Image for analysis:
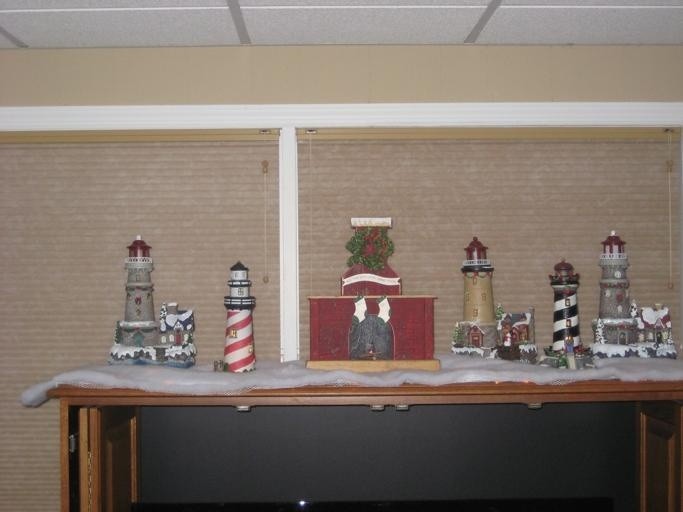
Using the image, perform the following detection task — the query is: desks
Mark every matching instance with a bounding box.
[45,379,683,512]
